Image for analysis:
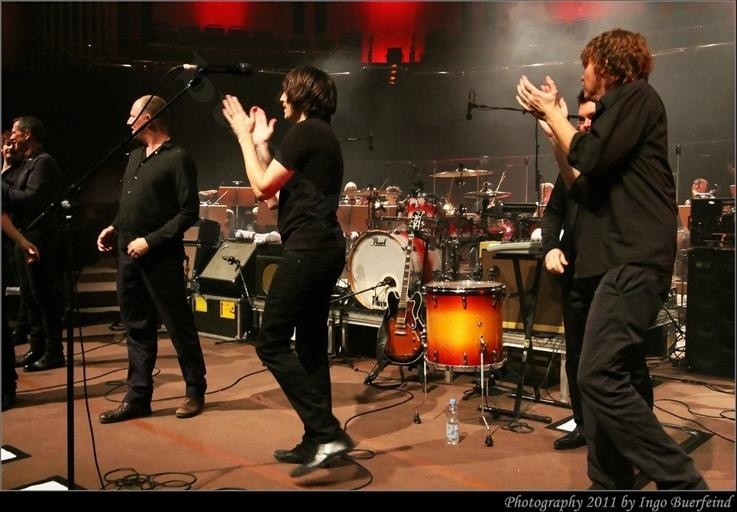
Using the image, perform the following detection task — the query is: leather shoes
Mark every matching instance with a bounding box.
[274,443,329,468]
[99,401,151,423]
[553,431,587,450]
[12,331,27,346]
[15,349,37,367]
[23,353,65,372]
[176,397,204,417]
[289,434,355,477]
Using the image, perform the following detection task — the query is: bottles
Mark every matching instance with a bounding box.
[447,399,459,445]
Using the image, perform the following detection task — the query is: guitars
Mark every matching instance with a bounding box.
[377,210,425,365]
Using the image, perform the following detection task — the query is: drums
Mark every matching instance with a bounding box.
[477,212,543,242]
[441,216,479,241]
[422,281,508,372]
[400,195,446,229]
[348,229,443,310]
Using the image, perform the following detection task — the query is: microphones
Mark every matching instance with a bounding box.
[183,62,253,75]
[368,130,374,151]
[466,93,472,120]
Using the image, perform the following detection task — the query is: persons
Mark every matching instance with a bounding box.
[1,116,66,412]
[675,162,735,313]
[340,181,557,246]
[96,95,207,424]
[542,89,597,450]
[514,28,709,492]
[197,186,282,245]
[220,65,354,478]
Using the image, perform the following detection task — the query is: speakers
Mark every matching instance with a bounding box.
[184,217,349,358]
[476,241,564,335]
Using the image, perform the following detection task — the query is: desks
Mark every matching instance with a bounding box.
[493,250,573,424]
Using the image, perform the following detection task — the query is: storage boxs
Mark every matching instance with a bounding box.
[191,292,253,342]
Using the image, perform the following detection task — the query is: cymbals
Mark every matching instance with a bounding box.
[464,190,511,200]
[340,190,404,196]
[430,169,495,177]
